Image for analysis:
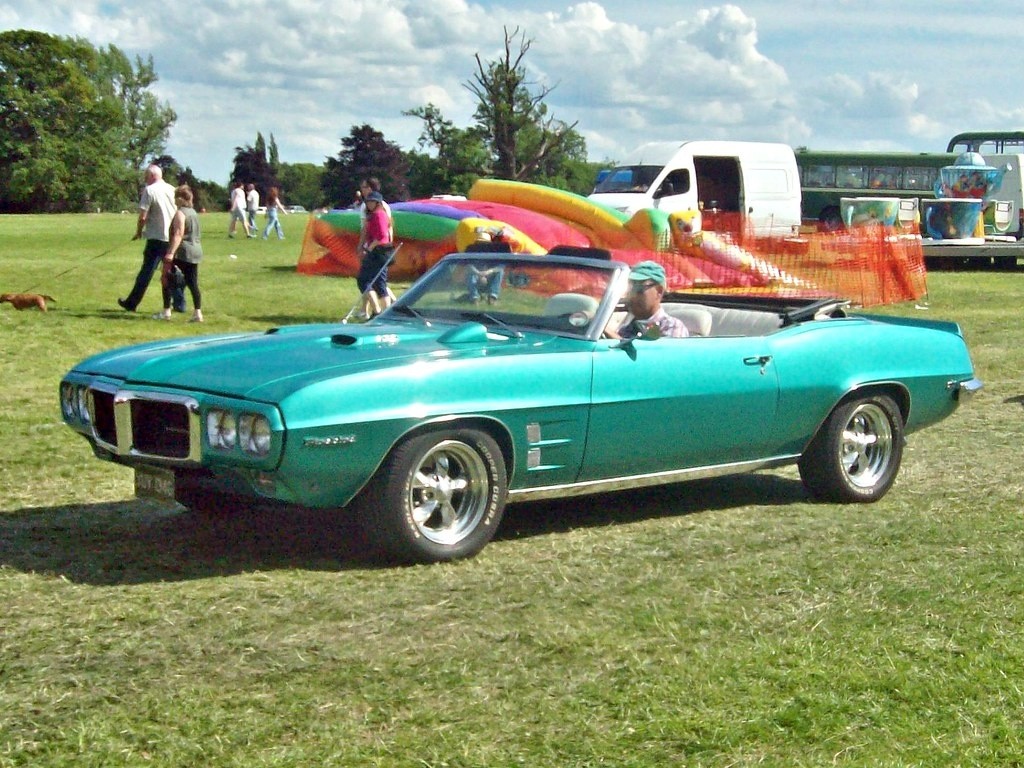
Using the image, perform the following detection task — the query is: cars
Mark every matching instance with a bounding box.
[57,239,985,559]
[256,207,267,215]
[284,205,310,214]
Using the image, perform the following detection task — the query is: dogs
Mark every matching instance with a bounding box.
[0,292,56,314]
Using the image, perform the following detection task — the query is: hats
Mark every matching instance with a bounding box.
[475,232,492,243]
[629,260,666,288]
[364,191,383,202]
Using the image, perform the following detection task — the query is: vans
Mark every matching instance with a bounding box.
[981,153,1024,238]
[430,195,466,202]
[582,139,805,253]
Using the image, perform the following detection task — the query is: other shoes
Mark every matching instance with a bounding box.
[118,297,135,312]
[354,311,369,320]
[228,234,234,238]
[246,235,254,237]
[152,312,170,320]
[186,316,203,322]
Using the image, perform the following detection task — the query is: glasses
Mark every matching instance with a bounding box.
[633,283,657,294]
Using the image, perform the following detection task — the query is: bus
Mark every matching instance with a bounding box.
[792,148,964,233]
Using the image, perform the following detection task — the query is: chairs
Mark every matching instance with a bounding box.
[543,293,599,319]
[667,309,711,337]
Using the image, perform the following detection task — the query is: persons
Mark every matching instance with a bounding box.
[346,177,396,320]
[228,182,258,238]
[464,233,513,307]
[152,185,204,322]
[246,184,259,232]
[356,191,391,326]
[117,164,178,312]
[261,187,287,240]
[582,260,689,338]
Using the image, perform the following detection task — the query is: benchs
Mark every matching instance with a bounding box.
[658,302,785,338]
